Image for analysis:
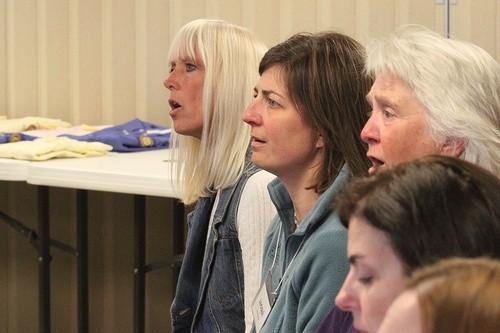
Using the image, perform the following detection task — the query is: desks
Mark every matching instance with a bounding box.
[0,124,186,333]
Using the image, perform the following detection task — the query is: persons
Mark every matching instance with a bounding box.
[333,153,500,333]
[162,18,270,333]
[376,256,500,333]
[242,31,377,333]
[361,24,500,179]
